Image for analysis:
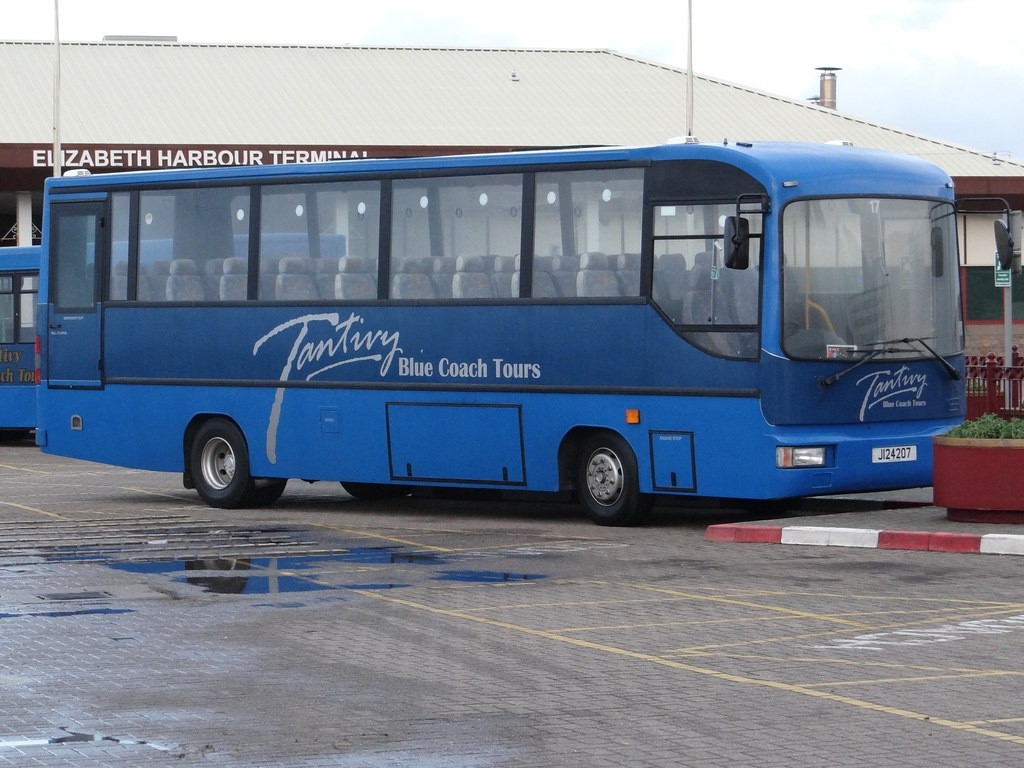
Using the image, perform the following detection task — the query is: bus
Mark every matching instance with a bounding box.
[32,139,1015,528]
[0,234,347,428]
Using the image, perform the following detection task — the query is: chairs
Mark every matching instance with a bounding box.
[114,251,780,368]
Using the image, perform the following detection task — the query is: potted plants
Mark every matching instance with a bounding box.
[932,411,1024,523]
